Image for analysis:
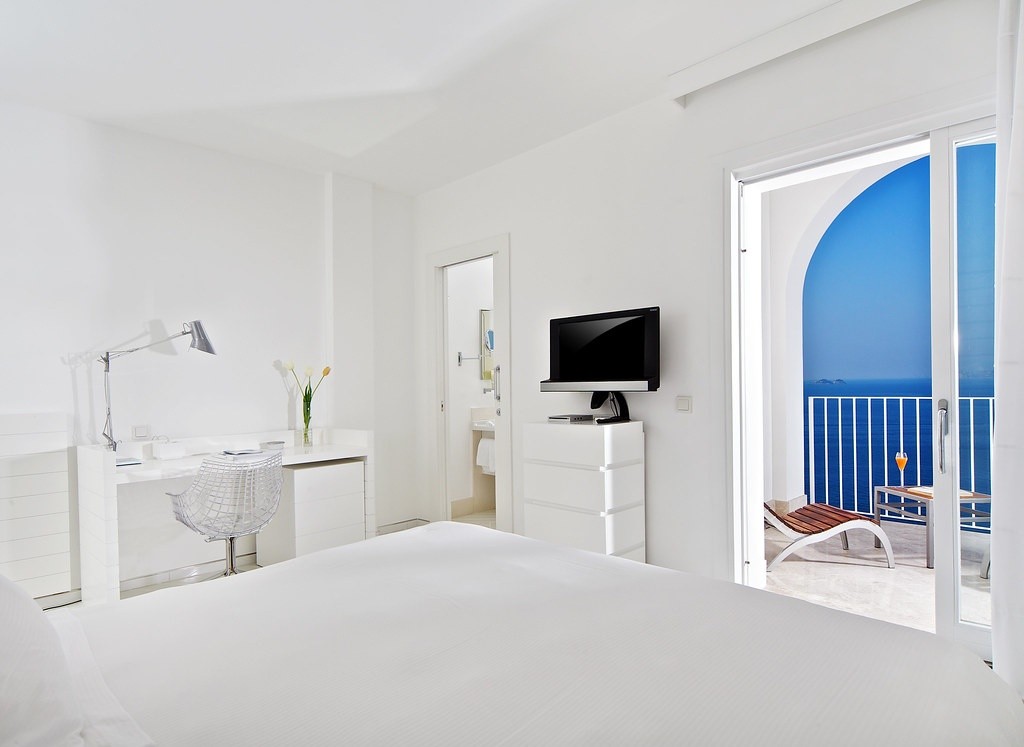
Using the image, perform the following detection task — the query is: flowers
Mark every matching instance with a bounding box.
[283,360,335,442]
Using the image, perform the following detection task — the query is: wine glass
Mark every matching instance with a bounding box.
[895,452,908,488]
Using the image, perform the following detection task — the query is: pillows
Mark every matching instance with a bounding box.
[0,574,156,747]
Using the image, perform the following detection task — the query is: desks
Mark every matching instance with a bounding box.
[77,428,376,606]
[871,485,991,568]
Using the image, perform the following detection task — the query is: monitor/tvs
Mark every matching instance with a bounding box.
[548,306,661,389]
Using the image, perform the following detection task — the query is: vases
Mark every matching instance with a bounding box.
[303,427,313,447]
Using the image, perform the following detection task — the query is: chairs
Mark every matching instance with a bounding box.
[764,501,898,572]
[163,451,286,583]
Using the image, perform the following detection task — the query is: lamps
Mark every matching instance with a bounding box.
[99,316,223,454]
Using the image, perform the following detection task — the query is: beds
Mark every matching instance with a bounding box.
[0,519,1024,747]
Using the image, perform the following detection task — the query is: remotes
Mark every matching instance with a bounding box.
[596,416,632,424]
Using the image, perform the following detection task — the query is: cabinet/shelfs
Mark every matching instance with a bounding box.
[520,417,648,562]
[253,458,364,564]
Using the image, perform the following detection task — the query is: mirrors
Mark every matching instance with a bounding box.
[479,309,494,381]
